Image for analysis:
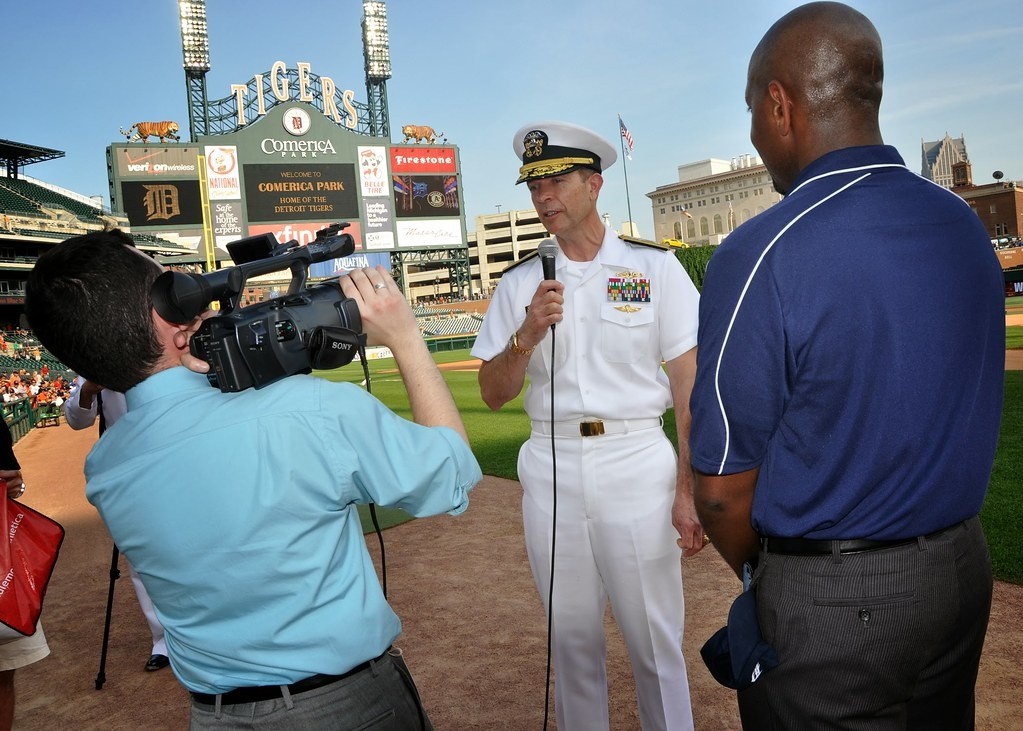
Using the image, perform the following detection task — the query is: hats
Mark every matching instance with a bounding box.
[512,121,618,184]
[10,389,14,393]
[14,381,19,385]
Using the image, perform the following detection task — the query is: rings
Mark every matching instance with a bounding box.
[375,283,385,290]
[703,534,711,546]
[21,484,25,492]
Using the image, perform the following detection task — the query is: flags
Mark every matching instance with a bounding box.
[619,117,634,152]
[683,211,693,218]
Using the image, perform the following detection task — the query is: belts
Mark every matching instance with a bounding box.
[760,533,916,555]
[192,645,396,706]
[531,418,660,437]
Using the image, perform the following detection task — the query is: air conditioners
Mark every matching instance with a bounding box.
[1007,180,1016,189]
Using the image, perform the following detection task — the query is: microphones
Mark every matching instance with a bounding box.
[538,239,559,329]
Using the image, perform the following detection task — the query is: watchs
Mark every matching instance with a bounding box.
[508,333,538,355]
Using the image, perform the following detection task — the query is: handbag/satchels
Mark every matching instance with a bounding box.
[0,478,67,635]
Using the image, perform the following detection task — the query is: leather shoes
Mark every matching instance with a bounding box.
[145,654,170,671]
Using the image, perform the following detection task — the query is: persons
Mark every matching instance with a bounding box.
[0,328,43,361]
[471,121,699,731]
[0,415,24,731]
[477,291,481,299]
[691,0,1006,730]
[4,212,11,229]
[0,365,77,425]
[24,232,486,731]
[418,296,451,307]
